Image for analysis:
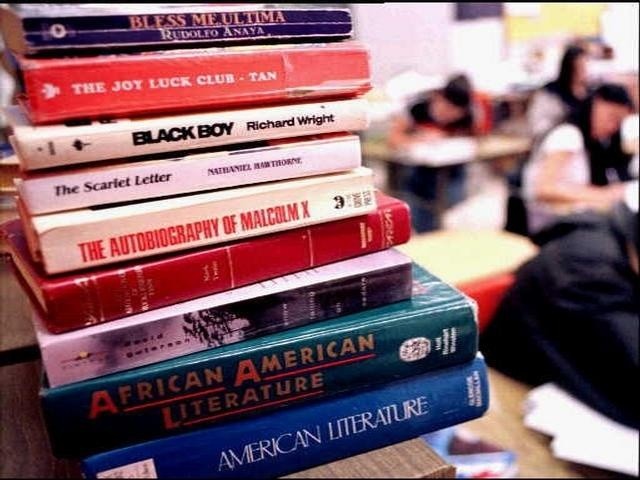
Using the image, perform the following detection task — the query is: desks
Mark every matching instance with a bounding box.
[278,437,456,480]
[364,134,533,226]
[0,260,87,480]
[391,227,540,328]
[427,369,637,480]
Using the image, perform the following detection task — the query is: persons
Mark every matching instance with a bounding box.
[526,47,590,146]
[504,84,639,247]
[478,198,638,429]
